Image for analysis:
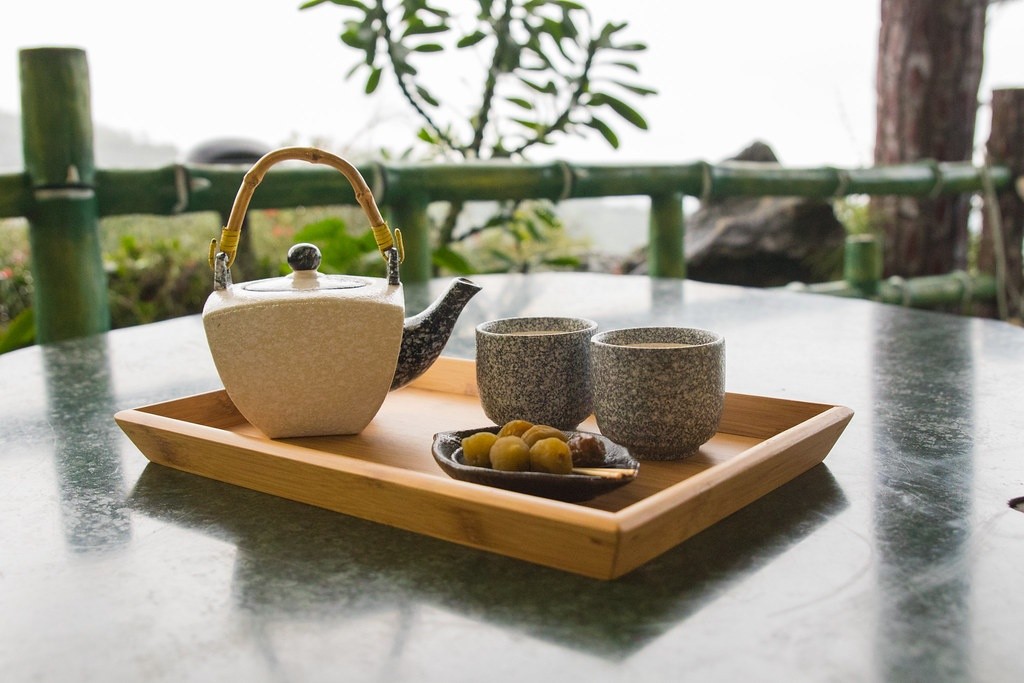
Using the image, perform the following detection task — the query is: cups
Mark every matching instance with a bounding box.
[591,326,726,463]
[475,316,599,431]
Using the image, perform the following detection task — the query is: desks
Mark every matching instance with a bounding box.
[0,269,1024,683]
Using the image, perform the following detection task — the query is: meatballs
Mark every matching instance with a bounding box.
[460,419,607,475]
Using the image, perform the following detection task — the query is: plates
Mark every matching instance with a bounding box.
[431,426,639,504]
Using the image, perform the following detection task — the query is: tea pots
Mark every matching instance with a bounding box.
[201,146,482,440]
